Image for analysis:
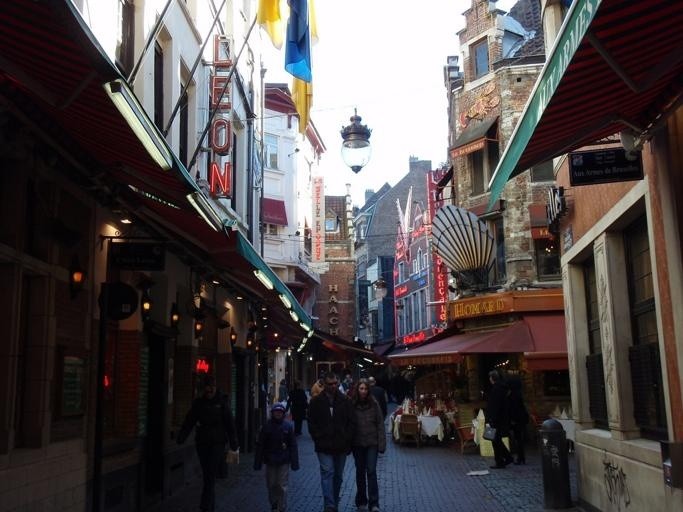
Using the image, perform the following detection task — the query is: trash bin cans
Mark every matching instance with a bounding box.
[538,419,572,510]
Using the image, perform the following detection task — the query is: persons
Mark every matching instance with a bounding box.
[277,374,388,435]
[254,402,299,511]
[350,377,386,511]
[177,380,240,511]
[480,369,514,470]
[507,377,530,464]
[305,372,358,512]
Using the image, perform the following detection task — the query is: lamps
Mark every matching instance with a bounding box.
[171,301,180,327]
[141,290,152,322]
[230,107,373,173]
[230,326,237,344]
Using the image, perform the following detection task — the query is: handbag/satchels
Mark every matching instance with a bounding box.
[226,449,240,465]
[482,421,497,441]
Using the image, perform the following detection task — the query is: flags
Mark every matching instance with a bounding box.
[255,0,322,138]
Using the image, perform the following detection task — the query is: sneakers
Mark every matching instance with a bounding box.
[270,501,380,512]
[489,452,527,469]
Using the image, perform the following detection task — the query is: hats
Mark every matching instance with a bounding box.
[269,403,286,413]
